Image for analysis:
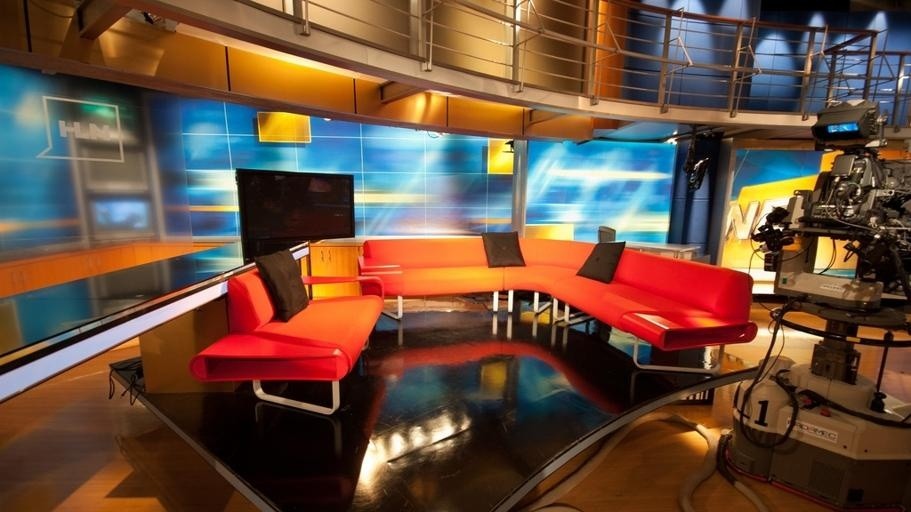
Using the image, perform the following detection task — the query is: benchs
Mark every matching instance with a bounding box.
[188,268,385,416]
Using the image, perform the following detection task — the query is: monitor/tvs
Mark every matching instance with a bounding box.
[237,169,355,265]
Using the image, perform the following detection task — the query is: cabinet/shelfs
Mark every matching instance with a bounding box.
[310,241,364,301]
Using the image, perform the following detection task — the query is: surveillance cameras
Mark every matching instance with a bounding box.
[682,135,710,193]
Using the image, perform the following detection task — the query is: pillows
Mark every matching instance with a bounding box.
[481,232,526,267]
[254,249,309,322]
